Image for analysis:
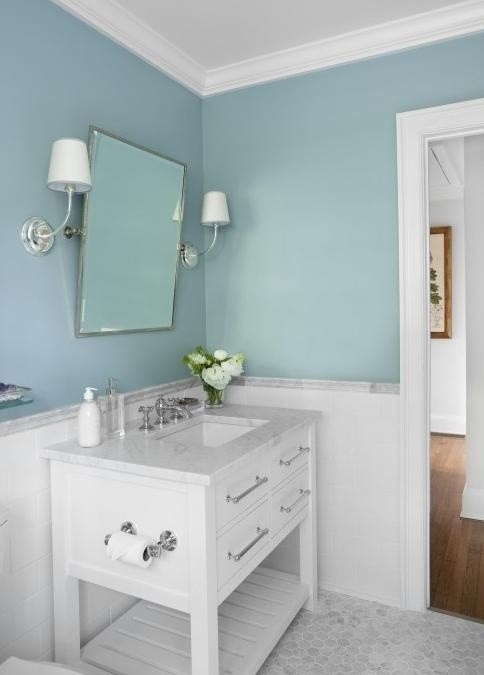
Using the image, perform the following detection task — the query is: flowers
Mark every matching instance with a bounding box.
[181,345,247,403]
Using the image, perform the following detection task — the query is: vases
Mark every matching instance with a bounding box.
[201,380,224,410]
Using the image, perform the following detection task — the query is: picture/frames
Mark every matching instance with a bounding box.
[429,228,454,342]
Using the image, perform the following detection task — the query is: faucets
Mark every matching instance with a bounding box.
[154,398,192,425]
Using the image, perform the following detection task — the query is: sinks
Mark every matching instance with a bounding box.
[141,413,272,449]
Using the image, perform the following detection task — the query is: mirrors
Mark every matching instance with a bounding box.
[78,123,187,338]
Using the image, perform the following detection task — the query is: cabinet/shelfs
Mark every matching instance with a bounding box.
[38,403,323,675]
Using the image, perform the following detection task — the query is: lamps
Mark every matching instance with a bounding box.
[180,190,230,271]
[20,137,92,255]
